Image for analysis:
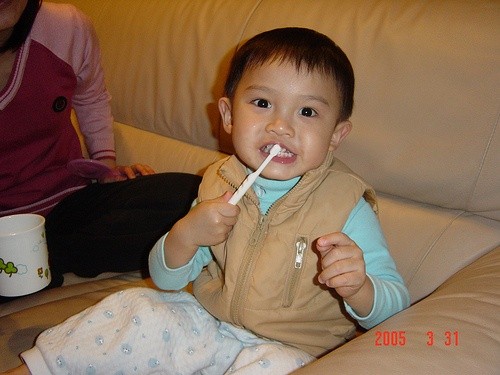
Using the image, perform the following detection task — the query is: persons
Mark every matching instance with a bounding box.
[0,0,202,302]
[0,27,411,375]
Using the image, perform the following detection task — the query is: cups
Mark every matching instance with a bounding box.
[0,214,53,298]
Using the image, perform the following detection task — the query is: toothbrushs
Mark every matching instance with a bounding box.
[227,144,282,207]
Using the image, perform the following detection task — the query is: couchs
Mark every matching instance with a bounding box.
[0,0,499,373]
